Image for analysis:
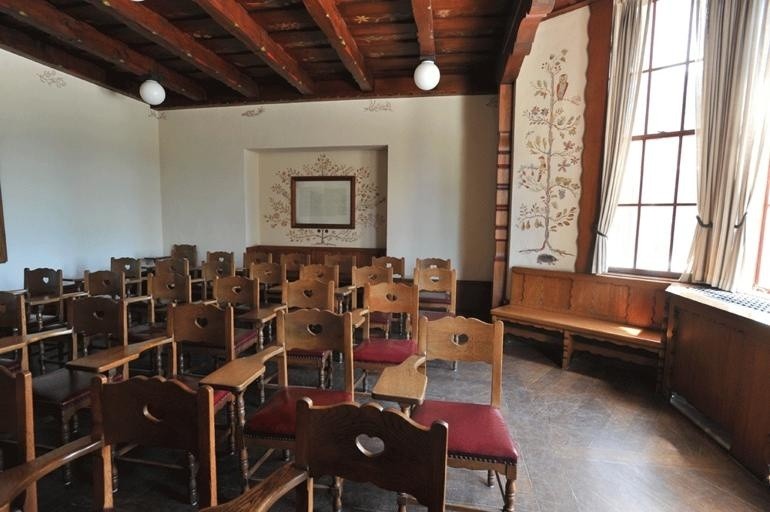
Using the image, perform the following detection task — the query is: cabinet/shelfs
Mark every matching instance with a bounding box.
[664,284,770,493]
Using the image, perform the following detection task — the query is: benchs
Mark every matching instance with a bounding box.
[487,264,674,373]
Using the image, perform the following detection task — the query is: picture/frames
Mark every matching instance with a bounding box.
[290,175,356,230]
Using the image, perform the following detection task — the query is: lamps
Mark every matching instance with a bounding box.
[140,69,168,106]
[413,53,441,90]
